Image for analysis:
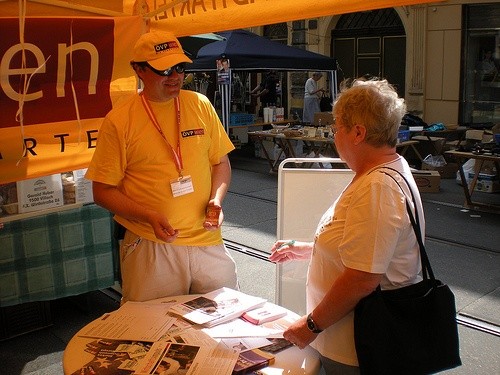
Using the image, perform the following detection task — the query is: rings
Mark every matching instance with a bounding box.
[294,344,296,346]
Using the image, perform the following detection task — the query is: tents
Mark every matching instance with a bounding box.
[184,29,337,134]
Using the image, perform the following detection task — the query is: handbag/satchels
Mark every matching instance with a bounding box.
[321,92,332,111]
[354,279,461,375]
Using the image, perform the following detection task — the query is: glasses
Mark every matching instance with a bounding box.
[143,62,186,77]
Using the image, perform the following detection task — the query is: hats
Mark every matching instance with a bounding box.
[129,32,193,70]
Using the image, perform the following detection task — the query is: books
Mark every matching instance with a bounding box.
[233,349,275,375]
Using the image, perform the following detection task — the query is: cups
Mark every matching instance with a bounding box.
[324,132,329,137]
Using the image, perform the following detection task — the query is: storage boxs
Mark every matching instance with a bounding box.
[230,107,333,162]
[398,124,500,194]
[0,300,57,343]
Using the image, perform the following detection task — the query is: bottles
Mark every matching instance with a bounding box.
[264,102,284,123]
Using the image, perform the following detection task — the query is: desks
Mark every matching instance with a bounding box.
[230,120,291,128]
[250,130,424,174]
[63,293,322,375]
[425,125,468,156]
[443,149,500,210]
[0,203,121,309]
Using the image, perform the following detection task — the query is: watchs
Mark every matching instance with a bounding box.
[306,313,323,333]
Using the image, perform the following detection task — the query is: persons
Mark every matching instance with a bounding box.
[303,72,324,123]
[269,77,426,375]
[84,31,237,305]
[220,60,229,73]
[155,357,192,375]
[249,72,277,107]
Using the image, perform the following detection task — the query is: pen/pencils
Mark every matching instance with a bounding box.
[278,240,295,249]
